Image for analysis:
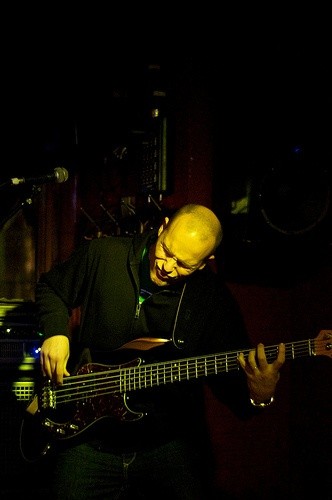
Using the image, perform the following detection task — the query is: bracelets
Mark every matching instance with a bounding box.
[248,394,274,409]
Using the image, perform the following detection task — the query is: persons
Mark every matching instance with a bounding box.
[34,203,287,500]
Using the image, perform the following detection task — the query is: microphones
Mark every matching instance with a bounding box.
[7,167,68,187]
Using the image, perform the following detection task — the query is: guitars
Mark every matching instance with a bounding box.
[20,329,332,464]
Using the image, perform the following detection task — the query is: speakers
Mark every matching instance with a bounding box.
[203,0,330,257]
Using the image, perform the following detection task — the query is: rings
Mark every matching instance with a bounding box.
[251,366,258,371]
